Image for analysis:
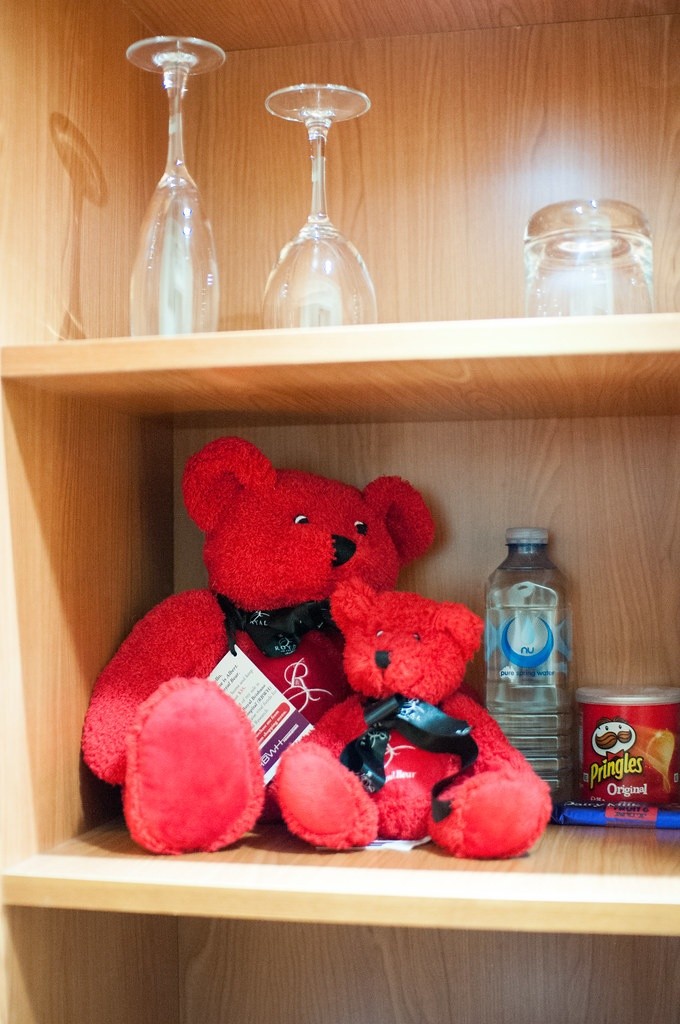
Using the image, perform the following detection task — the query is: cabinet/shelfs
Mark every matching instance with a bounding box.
[0,0,680,1024]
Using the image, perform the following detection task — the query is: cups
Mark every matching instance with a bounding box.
[526,200,654,313]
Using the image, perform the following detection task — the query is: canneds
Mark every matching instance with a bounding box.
[573,683,680,809]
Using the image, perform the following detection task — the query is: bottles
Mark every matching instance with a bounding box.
[484,528,575,816]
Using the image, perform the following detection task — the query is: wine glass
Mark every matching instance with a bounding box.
[126,37,226,335]
[264,84,377,328]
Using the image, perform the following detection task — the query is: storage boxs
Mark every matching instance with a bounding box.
[576,686,680,808]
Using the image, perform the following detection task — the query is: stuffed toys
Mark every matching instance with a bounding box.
[81,434,437,845]
[270,577,554,859]
